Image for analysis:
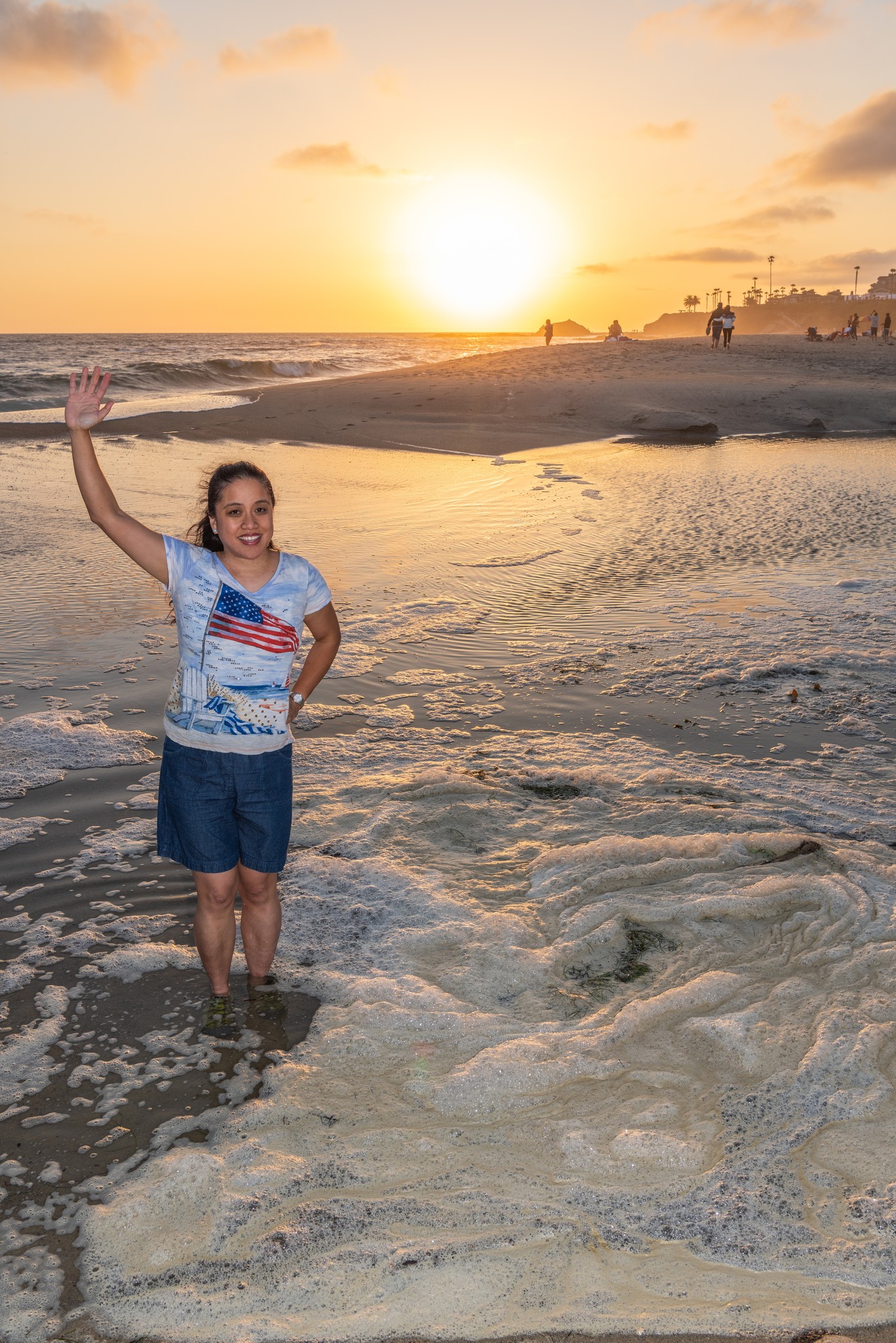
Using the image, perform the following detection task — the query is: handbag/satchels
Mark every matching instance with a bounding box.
[732,325,735,330]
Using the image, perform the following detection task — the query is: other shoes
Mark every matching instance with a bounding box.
[706,327,711,335]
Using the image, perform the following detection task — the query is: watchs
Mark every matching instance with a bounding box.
[288,691,304,709]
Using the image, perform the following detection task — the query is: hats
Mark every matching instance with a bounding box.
[717,302,723,307]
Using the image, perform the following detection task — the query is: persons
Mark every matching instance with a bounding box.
[881,313,891,342]
[544,319,553,346]
[63,365,342,994]
[707,302,735,349]
[847,313,860,341]
[870,309,879,341]
[713,305,736,349]
[605,320,624,341]
